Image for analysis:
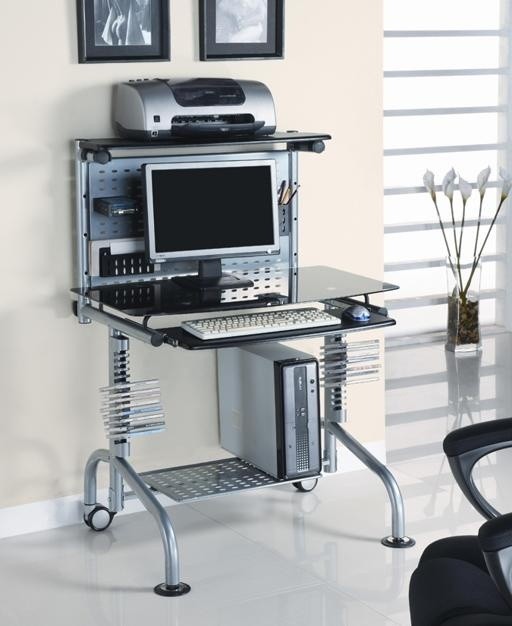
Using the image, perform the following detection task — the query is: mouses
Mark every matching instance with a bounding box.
[345,302,371,323]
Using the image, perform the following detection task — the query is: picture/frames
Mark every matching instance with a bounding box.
[76,0,285,64]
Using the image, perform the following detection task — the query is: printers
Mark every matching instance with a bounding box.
[109,76,277,143]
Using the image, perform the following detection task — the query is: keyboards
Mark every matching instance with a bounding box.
[182,307,342,341]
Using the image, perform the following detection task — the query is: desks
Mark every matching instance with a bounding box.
[72,263,415,596]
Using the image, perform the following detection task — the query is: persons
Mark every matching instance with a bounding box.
[104,0,141,45]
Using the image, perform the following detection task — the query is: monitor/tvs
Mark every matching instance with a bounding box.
[142,159,282,287]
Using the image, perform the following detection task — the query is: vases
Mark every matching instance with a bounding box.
[446,289,483,354]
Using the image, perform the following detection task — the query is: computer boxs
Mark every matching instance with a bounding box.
[212,343,322,481]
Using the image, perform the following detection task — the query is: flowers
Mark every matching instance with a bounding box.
[423,166,512,301]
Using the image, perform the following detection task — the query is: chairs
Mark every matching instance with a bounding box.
[409,417,512,626]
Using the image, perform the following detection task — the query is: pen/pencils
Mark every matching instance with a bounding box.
[278,180,300,206]
